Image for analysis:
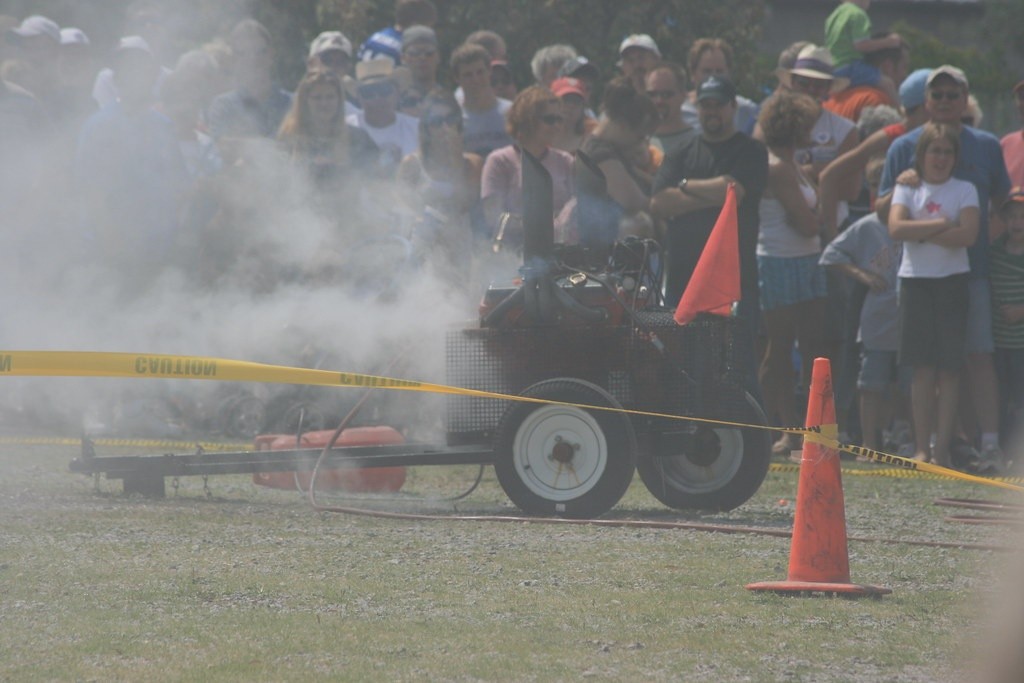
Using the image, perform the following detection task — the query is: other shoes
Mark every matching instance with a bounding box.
[977,444,1004,476]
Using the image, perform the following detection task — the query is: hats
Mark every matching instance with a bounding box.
[619,33,662,59]
[310,31,352,58]
[998,185,1024,214]
[9,16,61,43]
[61,28,90,46]
[899,68,933,110]
[695,75,735,104]
[571,56,598,79]
[776,43,850,96]
[121,36,150,54]
[341,60,414,100]
[925,64,969,88]
[552,78,587,100]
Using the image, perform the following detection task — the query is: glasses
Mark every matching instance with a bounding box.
[406,47,437,58]
[428,115,460,128]
[930,89,963,103]
[356,84,390,100]
[539,114,565,126]
[646,90,675,99]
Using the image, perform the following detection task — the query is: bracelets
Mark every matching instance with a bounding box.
[680,177,687,190]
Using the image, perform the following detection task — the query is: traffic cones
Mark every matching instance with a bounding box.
[745,354,899,596]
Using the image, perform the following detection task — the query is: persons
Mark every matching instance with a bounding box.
[0,0,1023,472]
[650,73,768,385]
[888,120,980,471]
